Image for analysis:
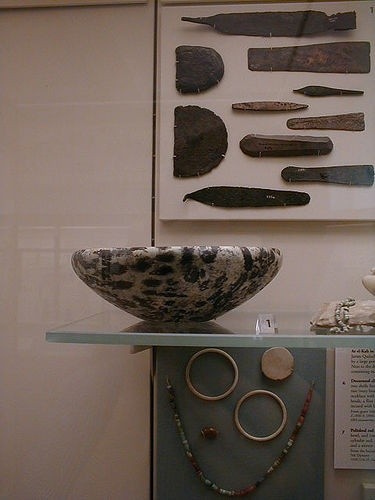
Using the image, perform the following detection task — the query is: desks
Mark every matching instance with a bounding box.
[45,309,375,349]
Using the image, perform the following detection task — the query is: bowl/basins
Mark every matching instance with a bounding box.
[71,246,283,323]
